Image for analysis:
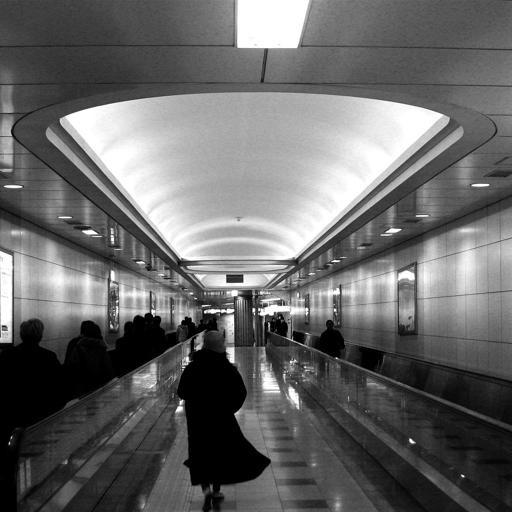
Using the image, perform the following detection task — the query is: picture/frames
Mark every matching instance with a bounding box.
[398,262,418,336]
[0,247,15,347]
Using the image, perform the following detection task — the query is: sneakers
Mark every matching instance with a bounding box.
[202,492,224,512]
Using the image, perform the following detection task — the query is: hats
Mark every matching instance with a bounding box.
[203,331,226,354]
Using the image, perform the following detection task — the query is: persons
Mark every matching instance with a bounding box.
[319,320,345,358]
[0,319,63,512]
[63,313,218,398]
[264,314,288,338]
[177,330,271,512]
